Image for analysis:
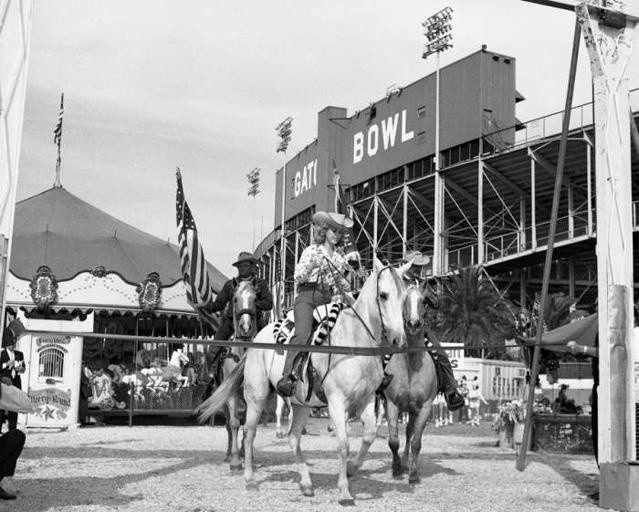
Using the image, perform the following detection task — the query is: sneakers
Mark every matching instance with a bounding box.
[1,487,17,501]
[277,376,294,397]
[444,390,465,411]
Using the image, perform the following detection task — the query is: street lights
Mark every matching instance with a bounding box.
[275,116,292,320]
[246,165,259,256]
[422,6,454,280]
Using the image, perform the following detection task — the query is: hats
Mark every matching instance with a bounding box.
[232,252,258,268]
[312,211,354,231]
[404,250,430,266]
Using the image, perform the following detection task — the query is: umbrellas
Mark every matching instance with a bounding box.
[53,108,65,145]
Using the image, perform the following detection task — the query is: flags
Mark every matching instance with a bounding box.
[174,179,221,332]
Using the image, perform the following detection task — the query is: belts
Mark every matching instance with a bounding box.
[301,282,333,291]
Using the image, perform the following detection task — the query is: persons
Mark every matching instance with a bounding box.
[276,211,355,395]
[1,337,26,435]
[203,250,274,371]
[457,374,490,427]
[0,317,30,498]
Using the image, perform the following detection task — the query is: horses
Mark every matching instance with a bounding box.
[193,254,416,507]
[382,273,439,486]
